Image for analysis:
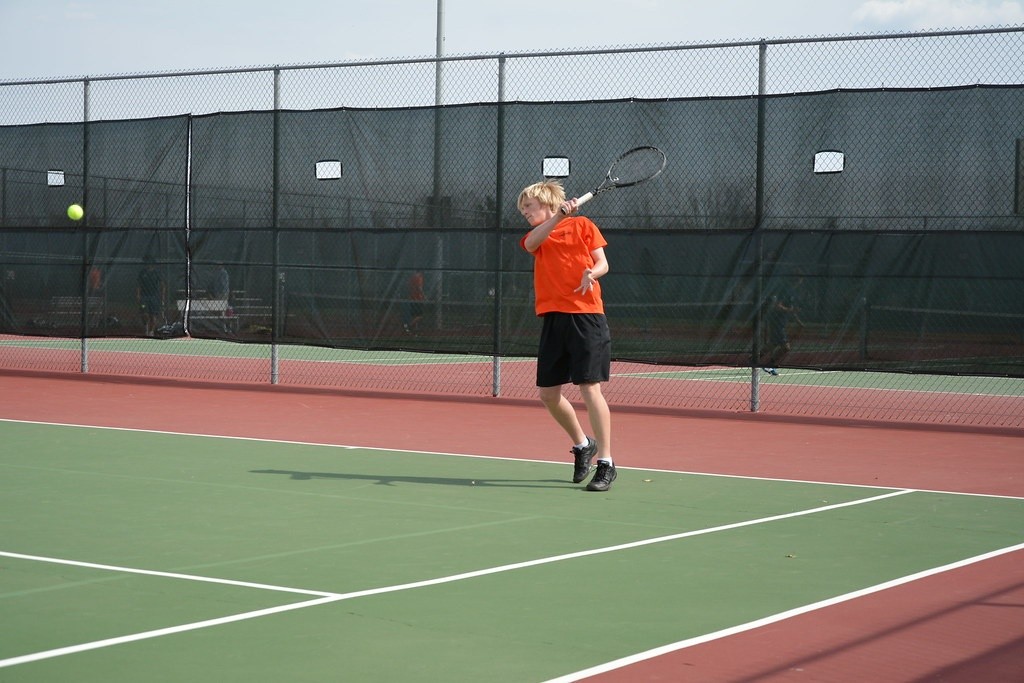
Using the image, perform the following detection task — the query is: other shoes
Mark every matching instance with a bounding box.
[403,322,411,336]
[415,333,422,338]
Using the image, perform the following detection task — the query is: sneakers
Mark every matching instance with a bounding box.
[569,435,598,483]
[763,367,778,376]
[586,460,617,491]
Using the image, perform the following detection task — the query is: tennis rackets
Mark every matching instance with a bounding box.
[561,145,666,216]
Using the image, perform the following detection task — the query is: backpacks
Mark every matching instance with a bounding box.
[153,319,186,341]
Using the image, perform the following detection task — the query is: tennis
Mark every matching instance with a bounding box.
[67,205,84,220]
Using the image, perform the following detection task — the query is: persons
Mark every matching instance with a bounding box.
[91,265,107,295]
[517,180,617,490]
[137,256,166,337]
[214,264,230,303]
[403,267,426,338]
[756,247,804,367]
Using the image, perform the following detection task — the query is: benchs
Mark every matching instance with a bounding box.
[35,295,103,334]
[174,290,239,336]
